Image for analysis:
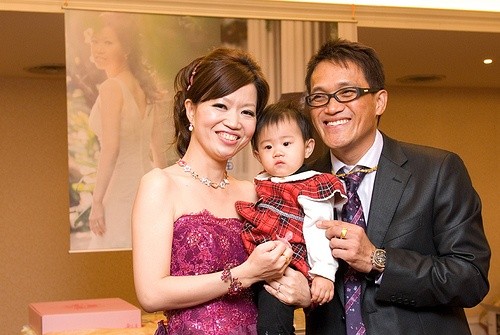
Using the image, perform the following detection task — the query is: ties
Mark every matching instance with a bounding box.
[337,166,370,334]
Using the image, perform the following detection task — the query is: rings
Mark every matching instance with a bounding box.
[282,252,290,264]
[276,283,283,292]
[340,227,348,239]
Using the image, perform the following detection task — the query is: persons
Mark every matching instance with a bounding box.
[88,13,165,251]
[297,39,492,335]
[132,50,312,334]
[236,101,346,334]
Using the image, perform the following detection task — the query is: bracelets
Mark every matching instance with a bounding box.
[220,259,250,296]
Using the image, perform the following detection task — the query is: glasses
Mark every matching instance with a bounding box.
[305,86,383,107]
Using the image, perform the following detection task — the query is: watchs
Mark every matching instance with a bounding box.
[364,247,387,287]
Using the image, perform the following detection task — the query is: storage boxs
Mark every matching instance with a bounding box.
[28,297,142,335]
